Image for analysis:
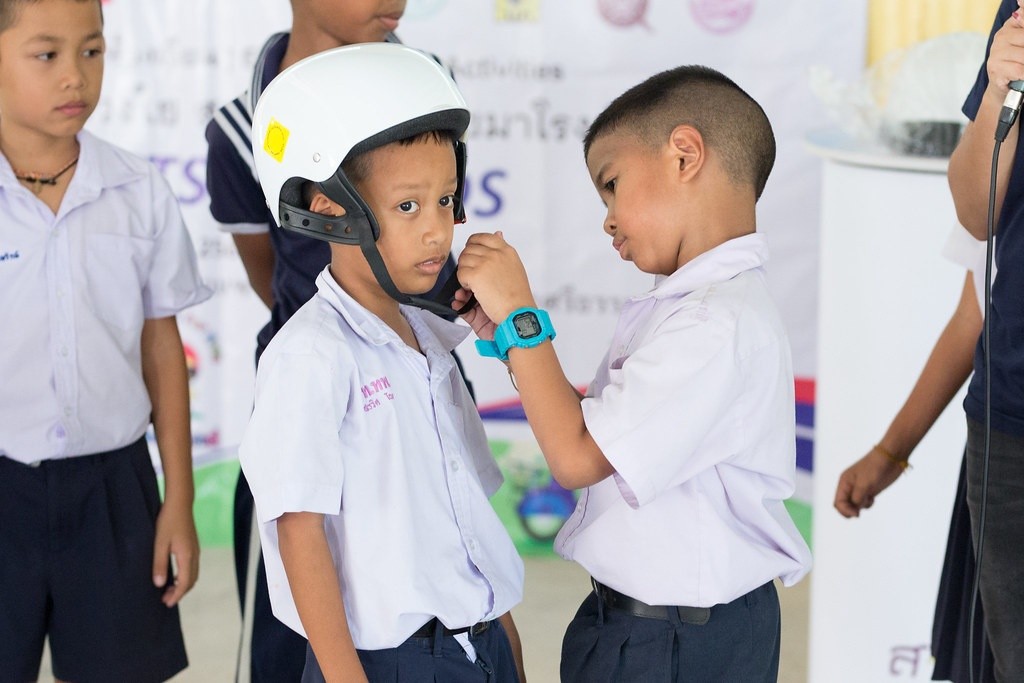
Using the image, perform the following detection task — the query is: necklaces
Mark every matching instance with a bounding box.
[17,153,78,193]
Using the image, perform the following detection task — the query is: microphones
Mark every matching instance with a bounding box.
[995,80,1024,142]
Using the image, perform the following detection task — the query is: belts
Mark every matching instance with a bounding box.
[590,575,710,626]
[409,617,490,639]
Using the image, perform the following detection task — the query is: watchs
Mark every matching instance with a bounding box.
[476,307,554,360]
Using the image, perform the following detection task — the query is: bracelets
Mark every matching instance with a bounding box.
[875,444,914,469]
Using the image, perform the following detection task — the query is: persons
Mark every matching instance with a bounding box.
[0,1,217,683]
[203,0,477,683]
[830,0,1024,683]
[450,62,814,683]
[238,41,530,683]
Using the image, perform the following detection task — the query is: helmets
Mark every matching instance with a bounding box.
[251,41,471,228]
